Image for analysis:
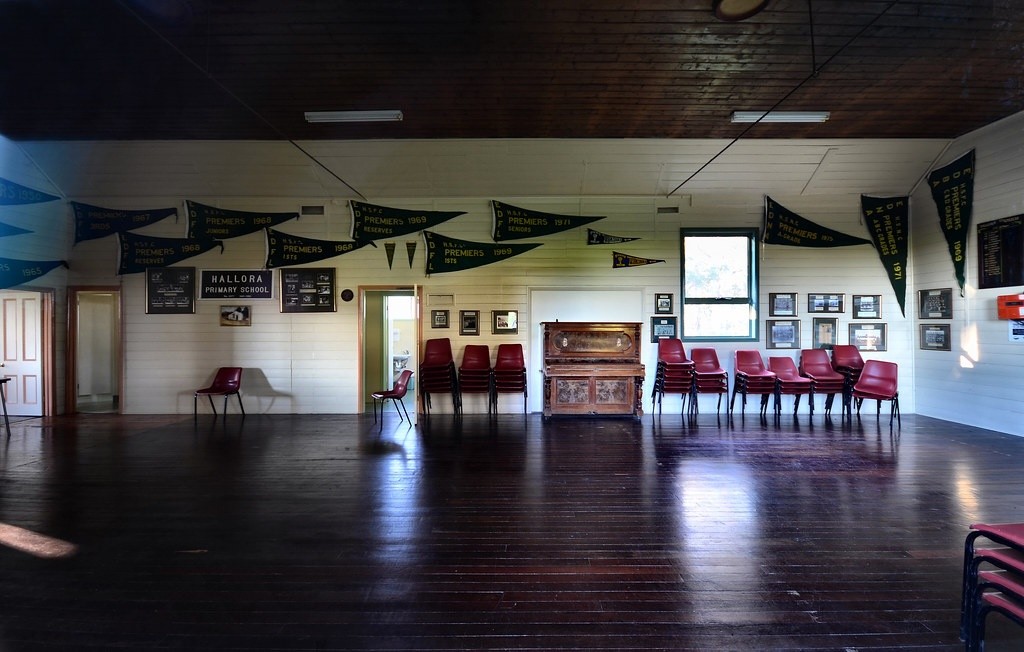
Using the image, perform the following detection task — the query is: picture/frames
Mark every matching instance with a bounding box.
[919,323,952,352]
[917,288,953,318]
[491,309,519,335]
[808,292,846,314]
[852,294,882,319]
[655,292,675,314]
[650,315,679,343]
[849,323,888,351]
[220,305,252,327]
[431,309,451,328]
[145,266,196,314]
[458,309,481,335]
[279,267,338,314]
[767,320,801,349]
[769,293,798,317]
[813,317,838,350]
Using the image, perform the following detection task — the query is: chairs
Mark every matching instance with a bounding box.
[372,370,414,427]
[955,522,1023,652]
[195,367,244,421]
[653,338,902,428]
[420,338,528,425]
